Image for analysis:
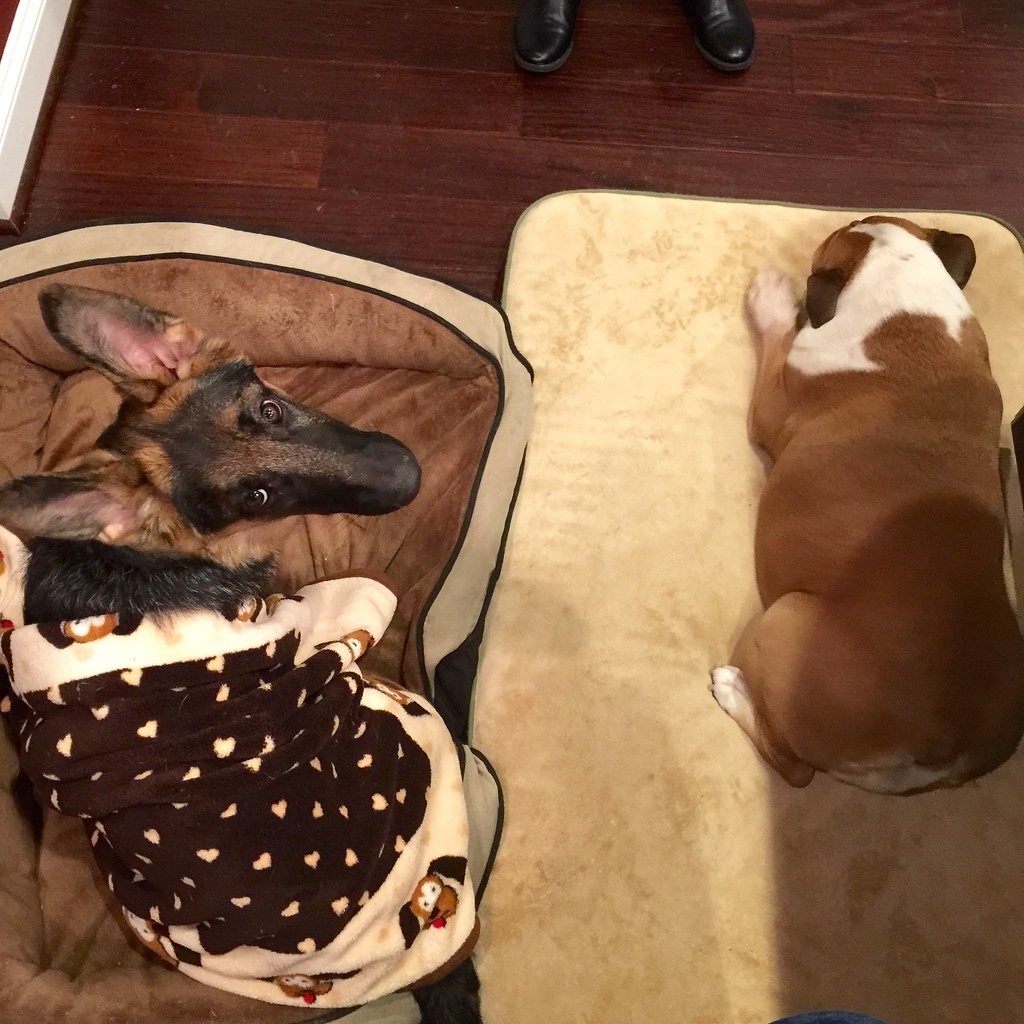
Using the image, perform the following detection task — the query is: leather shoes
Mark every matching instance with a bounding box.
[511,0,580,73]
[681,0,756,71]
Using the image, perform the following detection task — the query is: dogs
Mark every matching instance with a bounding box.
[0,285,423,626]
[703,212,1024,820]
